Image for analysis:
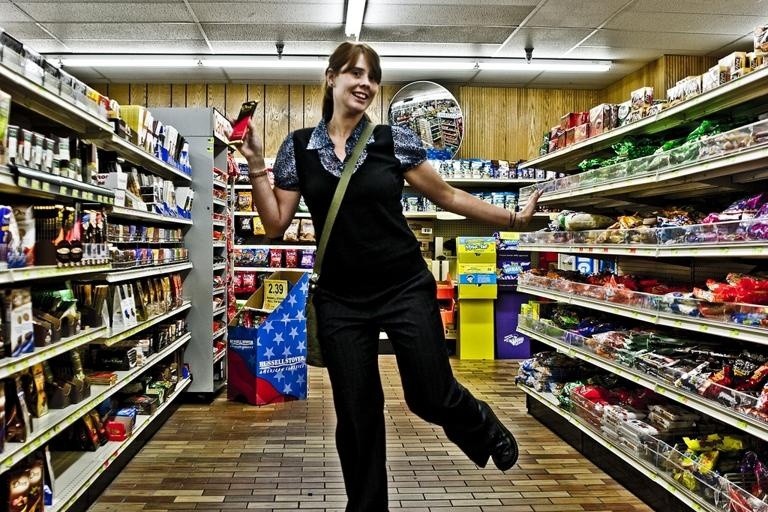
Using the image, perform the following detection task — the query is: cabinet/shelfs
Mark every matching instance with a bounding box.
[517,61,768,511]
[233,155,552,347]
[391,99,460,149]
[0,28,229,511]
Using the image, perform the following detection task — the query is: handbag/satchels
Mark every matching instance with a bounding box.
[306,283,326,368]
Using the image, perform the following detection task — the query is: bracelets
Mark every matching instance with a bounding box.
[250,167,269,179]
[509,210,517,229]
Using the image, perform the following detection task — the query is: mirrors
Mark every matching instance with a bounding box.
[387,80,466,160]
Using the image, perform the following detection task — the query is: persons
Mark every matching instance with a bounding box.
[222,41,549,511]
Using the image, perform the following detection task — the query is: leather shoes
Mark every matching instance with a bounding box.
[480,400,518,470]
